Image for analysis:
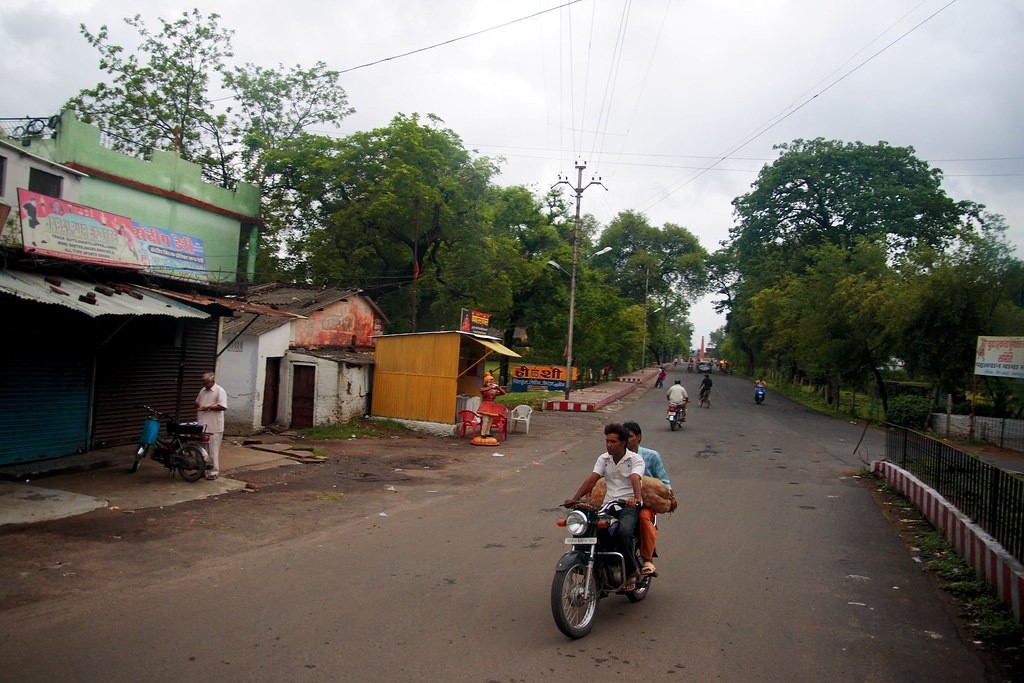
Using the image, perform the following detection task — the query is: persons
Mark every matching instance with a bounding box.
[623,420,673,575]
[689,358,694,363]
[655,369,666,387]
[666,379,689,422]
[699,374,713,398]
[564,423,646,592]
[674,356,678,366]
[192,371,228,480]
[461,312,472,332]
[755,376,766,387]
[600,365,610,383]
[476,371,505,439]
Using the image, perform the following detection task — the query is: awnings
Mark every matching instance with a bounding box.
[0,262,214,321]
[456,334,523,379]
[131,278,311,358]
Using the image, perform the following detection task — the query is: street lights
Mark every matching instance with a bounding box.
[662,318,675,363]
[641,307,662,374]
[670,333,682,362]
[548,247,614,400]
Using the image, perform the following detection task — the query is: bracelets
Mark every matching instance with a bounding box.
[208,408,210,411]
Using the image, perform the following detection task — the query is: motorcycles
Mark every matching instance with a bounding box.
[665,398,691,432]
[549,496,660,640]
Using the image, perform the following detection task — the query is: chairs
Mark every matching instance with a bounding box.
[459,401,533,444]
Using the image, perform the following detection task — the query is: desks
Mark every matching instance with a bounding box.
[456,396,481,423]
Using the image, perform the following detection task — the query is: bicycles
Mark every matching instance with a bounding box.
[131,404,214,482]
[697,385,712,409]
[657,373,664,389]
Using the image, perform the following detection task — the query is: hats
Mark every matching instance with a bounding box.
[703,374,709,377]
[675,379,681,384]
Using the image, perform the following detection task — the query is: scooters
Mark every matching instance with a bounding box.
[687,362,695,373]
[752,384,766,405]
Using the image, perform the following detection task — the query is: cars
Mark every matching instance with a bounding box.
[697,361,713,374]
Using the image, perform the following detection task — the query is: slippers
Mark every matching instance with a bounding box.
[641,561,656,574]
[621,578,637,592]
